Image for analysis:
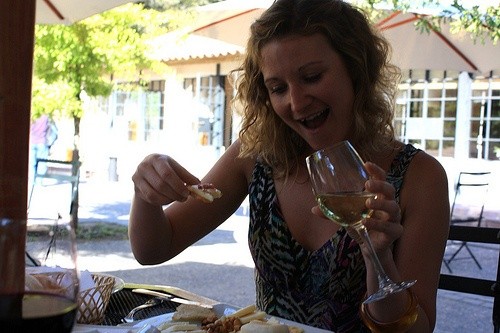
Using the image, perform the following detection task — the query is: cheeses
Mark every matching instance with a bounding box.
[229,303,266,323]
[161,321,208,333]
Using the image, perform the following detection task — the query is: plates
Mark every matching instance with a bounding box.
[94,272,124,293]
[133,304,334,333]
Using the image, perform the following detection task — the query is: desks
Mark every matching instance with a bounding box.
[98,284,334,333]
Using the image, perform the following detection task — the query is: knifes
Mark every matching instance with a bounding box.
[131,288,212,309]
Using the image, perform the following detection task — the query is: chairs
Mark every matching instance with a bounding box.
[437,227,500,333]
[24,158,82,265]
[443,172,492,272]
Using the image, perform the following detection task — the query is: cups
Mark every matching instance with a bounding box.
[0,216,80,333]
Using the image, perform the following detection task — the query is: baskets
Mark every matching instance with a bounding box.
[25,271,116,325]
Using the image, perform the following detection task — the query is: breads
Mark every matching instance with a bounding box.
[238,319,290,333]
[172,303,216,322]
[186,183,222,204]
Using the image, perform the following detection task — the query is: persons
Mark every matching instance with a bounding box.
[128,0,450,333]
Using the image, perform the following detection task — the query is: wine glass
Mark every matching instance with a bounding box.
[305,141,418,303]
